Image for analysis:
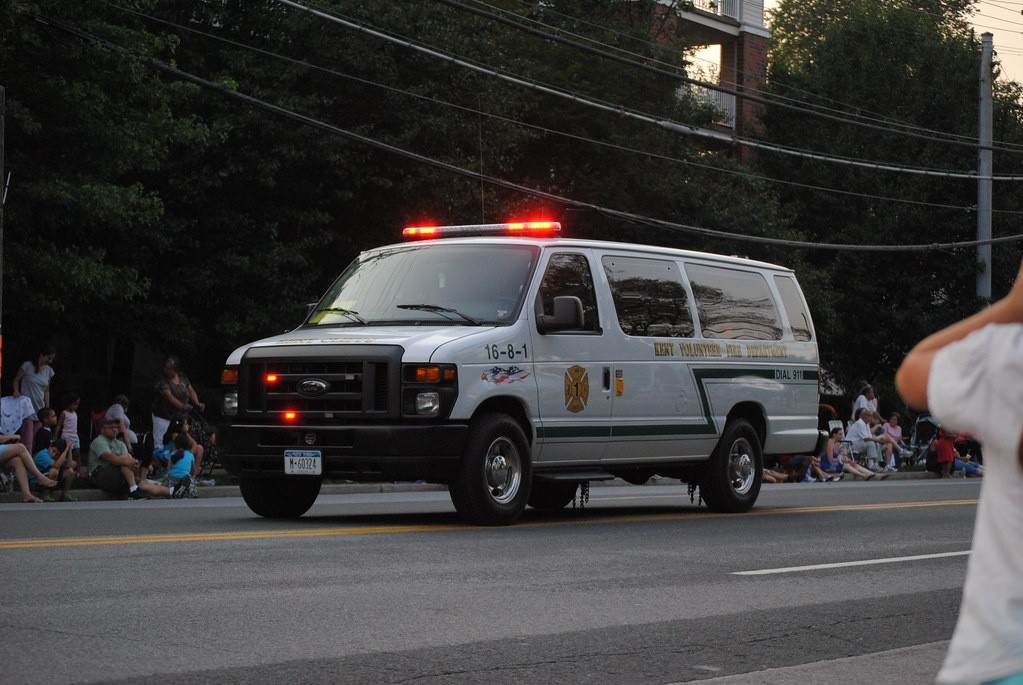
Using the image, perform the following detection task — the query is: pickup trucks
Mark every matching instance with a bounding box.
[215,221,821,523]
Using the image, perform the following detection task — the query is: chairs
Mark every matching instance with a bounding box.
[828,421,857,463]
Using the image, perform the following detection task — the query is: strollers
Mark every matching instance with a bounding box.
[180,409,235,485]
[906,414,941,465]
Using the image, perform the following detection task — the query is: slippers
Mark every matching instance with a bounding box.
[22,496,43,503]
[39,480,57,488]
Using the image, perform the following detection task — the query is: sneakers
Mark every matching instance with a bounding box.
[171,474,191,499]
[899,448,913,457]
[40,495,54,502]
[129,486,150,500]
[59,494,77,501]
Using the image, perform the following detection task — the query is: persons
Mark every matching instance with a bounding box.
[896,259,1023,685]
[154,356,204,422]
[925,425,984,478]
[12,345,55,457]
[55,393,82,466]
[760,380,914,481]
[0,407,78,502]
[87,395,204,501]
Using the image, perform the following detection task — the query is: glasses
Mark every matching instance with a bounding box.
[104,425,120,429]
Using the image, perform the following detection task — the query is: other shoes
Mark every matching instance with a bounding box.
[874,472,889,480]
[869,463,883,473]
[862,472,876,481]
[782,471,844,484]
[944,474,953,479]
[884,465,898,472]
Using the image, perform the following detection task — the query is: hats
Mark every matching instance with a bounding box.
[101,416,121,426]
